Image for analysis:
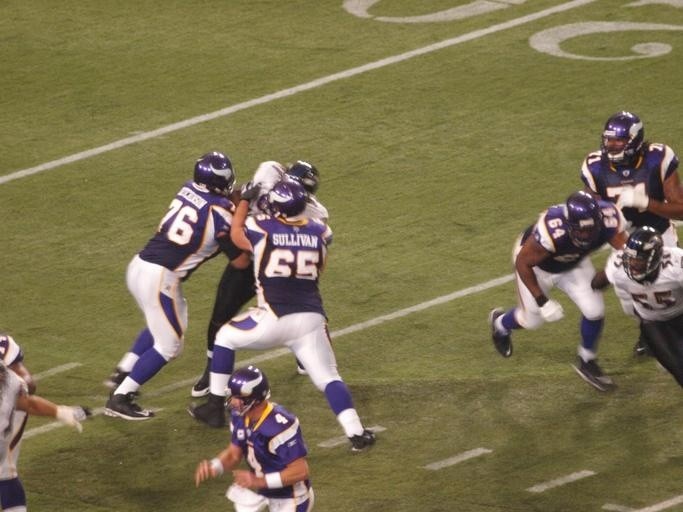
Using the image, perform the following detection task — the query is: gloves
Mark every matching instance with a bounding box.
[536,294,564,323]
[56,405,93,433]
[612,188,650,212]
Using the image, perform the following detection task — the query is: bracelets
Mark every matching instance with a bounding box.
[266,472,283,488]
[210,457,224,476]
[535,294,548,307]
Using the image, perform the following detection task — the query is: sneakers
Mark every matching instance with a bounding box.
[572,352,612,391]
[186,402,224,428]
[104,392,154,420]
[633,340,653,357]
[104,368,142,398]
[350,429,376,453]
[191,372,210,397]
[489,307,512,358]
[297,365,308,376]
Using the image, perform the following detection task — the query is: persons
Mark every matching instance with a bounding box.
[0,334,36,466]
[0,363,86,512]
[591,226,682,389]
[581,110,682,358]
[489,190,631,392]
[195,367,314,512]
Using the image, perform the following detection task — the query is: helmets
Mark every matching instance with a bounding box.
[224,366,271,416]
[567,190,604,244]
[600,110,644,166]
[194,153,319,219]
[622,226,664,282]
